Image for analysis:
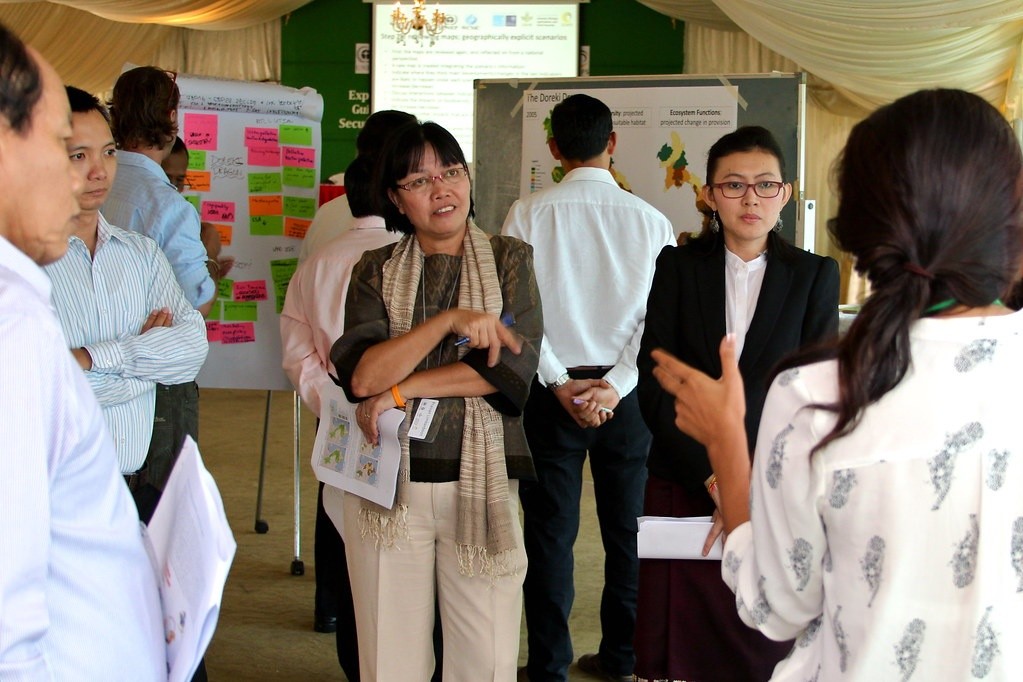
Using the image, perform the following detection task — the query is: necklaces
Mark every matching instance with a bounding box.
[924,295,1004,309]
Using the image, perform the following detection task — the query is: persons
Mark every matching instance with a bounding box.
[328,121,543,681]
[160,136,233,279]
[41,85,209,515]
[0,25,169,682]
[637,124,841,681]
[299,110,420,636]
[651,89,1022,682]
[501,93,679,682]
[97,66,221,529]
[277,156,443,681]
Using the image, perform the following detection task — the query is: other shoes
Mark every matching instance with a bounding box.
[314,615,337,633]
[576,654,635,682]
[517,665,531,682]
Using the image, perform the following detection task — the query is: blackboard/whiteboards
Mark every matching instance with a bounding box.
[472,70,807,250]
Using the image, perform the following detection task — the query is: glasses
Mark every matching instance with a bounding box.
[169,176,191,192]
[395,166,468,195]
[709,180,784,199]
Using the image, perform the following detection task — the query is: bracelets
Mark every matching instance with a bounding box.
[391,384,406,408]
[205,257,219,275]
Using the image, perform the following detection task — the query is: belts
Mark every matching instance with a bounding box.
[123,460,149,492]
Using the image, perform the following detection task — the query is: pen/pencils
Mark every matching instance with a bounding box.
[573,399,613,413]
[455,317,515,346]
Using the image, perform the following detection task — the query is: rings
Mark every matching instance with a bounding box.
[364,413,370,418]
